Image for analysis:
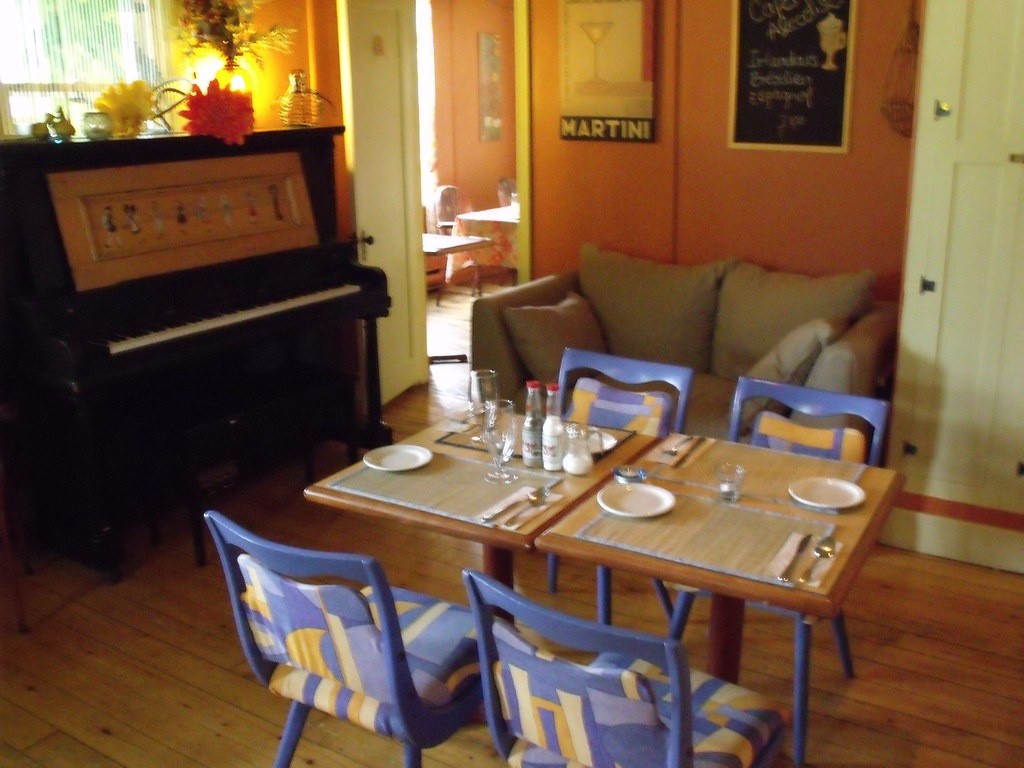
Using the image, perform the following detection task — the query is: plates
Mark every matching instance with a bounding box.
[588,430,618,453]
[788,476,866,508]
[597,483,676,517]
[363,445,433,471]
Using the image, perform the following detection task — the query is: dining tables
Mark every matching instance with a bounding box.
[304,408,904,686]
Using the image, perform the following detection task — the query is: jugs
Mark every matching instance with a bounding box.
[562,423,603,475]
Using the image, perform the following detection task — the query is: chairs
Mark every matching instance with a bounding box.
[667,375,887,768]
[462,567,787,768]
[547,346,692,623]
[433,185,483,306]
[203,509,484,768]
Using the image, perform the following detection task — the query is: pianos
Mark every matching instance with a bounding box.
[0,125,395,580]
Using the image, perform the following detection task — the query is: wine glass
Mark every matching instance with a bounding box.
[485,400,518,484]
[468,370,498,441]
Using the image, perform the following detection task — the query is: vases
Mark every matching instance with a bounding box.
[214,59,254,94]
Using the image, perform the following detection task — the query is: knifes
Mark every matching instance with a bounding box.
[481,488,533,521]
[671,437,706,468]
[778,534,813,581]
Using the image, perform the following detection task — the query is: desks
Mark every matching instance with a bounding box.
[445,205,520,287]
[421,233,495,257]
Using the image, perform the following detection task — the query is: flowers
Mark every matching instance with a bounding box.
[166,0,298,92]
[155,79,256,146]
[92,79,158,139]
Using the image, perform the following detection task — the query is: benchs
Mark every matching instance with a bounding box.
[144,363,361,568]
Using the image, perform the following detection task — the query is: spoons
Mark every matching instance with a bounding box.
[658,435,694,456]
[504,486,547,526]
[798,537,835,582]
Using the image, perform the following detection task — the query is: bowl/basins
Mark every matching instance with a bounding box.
[83,113,111,141]
[611,465,647,484]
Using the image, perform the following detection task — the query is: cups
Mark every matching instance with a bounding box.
[712,462,747,501]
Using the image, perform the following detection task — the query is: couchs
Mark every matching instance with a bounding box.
[471,244,897,447]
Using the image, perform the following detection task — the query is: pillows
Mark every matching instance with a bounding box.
[502,291,606,390]
[729,315,836,435]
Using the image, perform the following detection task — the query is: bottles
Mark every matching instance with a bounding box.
[523,380,562,471]
[511,193,520,219]
[281,70,322,127]
